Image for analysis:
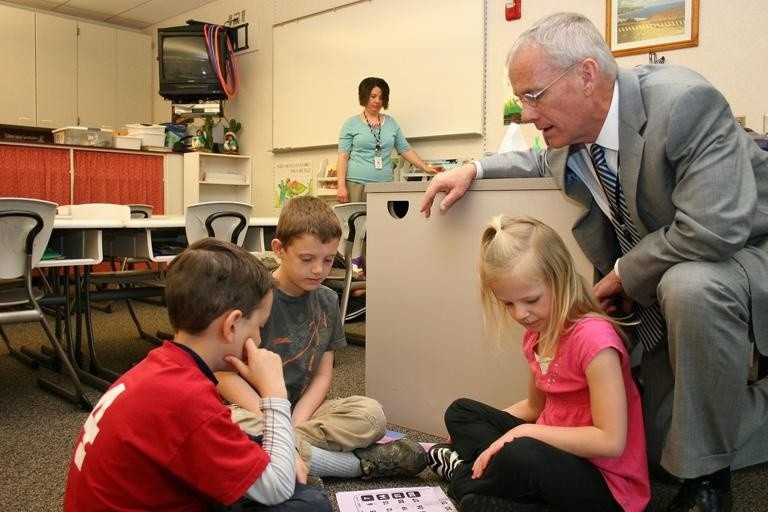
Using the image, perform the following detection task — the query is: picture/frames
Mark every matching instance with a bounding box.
[605,1,699,57]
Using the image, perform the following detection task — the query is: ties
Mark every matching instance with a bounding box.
[590,144,663,352]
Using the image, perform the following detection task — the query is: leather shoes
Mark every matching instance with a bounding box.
[664,477,733,511]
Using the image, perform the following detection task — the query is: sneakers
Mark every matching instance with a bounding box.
[354,439,429,482]
[426,442,462,481]
[460,493,550,512]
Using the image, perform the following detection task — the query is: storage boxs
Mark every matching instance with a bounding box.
[134,133,167,147]
[126,125,167,135]
[113,136,141,151]
[52,127,113,148]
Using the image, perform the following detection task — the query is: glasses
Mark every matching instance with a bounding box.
[513,62,583,109]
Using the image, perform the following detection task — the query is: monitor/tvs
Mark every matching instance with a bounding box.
[157,25,228,100]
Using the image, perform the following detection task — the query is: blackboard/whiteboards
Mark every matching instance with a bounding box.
[271,0,488,152]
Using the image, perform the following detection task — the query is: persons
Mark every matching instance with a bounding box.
[334,77,446,276]
[426,211,653,512]
[420,10,768,512]
[60,239,334,511]
[327,253,366,324]
[257,195,429,487]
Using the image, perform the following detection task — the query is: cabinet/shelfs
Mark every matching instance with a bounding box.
[316,156,476,205]
[365,177,599,439]
[77,21,153,130]
[183,151,252,216]
[0,144,167,278]
[0,4,78,129]
